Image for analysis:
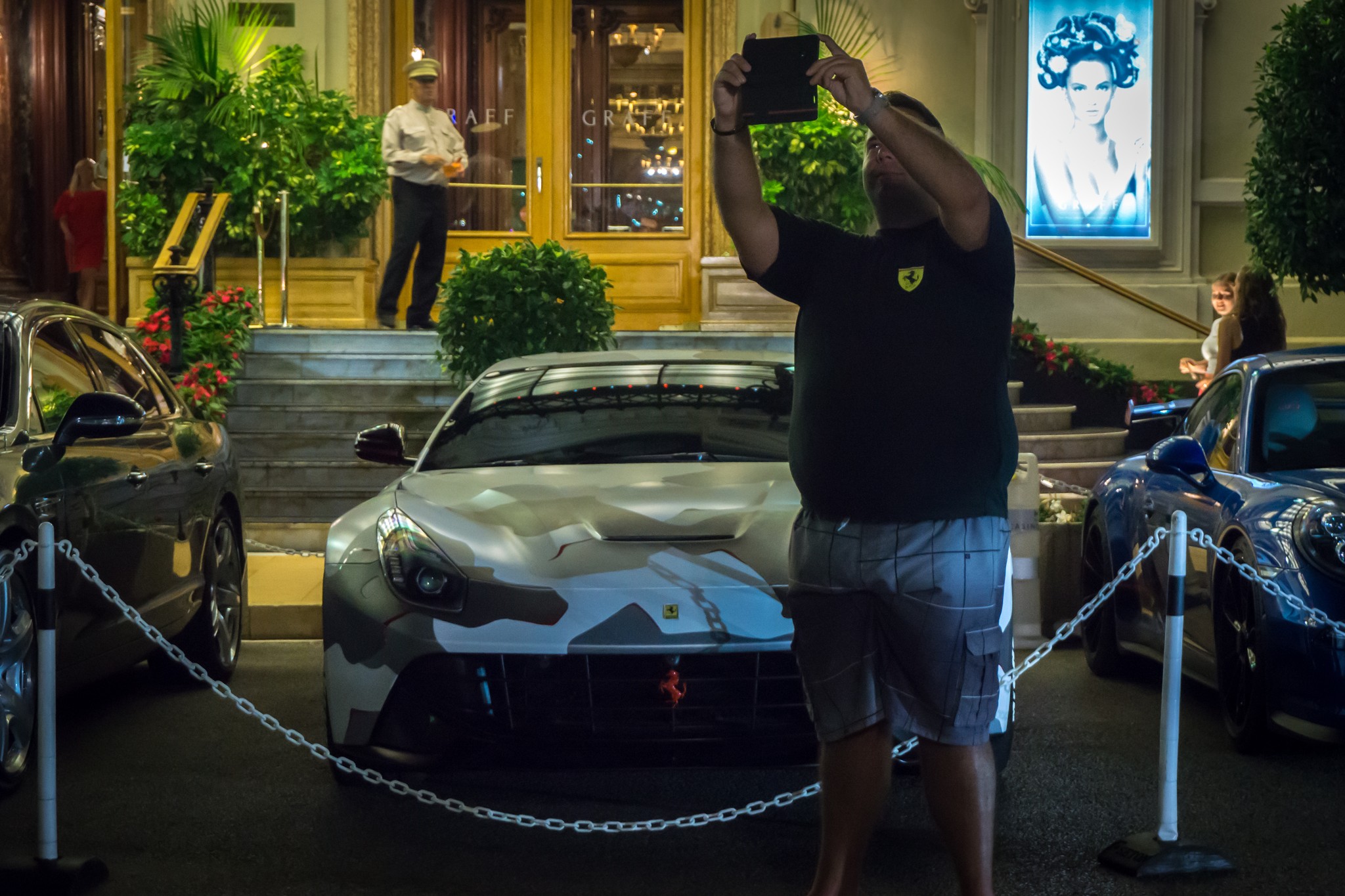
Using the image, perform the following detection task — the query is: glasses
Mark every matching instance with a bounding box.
[413,76,435,83]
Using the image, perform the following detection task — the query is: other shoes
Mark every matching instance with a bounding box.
[406,316,439,330]
[377,311,396,328]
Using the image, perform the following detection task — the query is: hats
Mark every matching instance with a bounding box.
[402,58,441,80]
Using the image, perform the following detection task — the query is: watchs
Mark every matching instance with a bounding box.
[852,87,890,124]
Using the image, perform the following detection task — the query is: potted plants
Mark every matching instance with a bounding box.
[1073,354,1137,427]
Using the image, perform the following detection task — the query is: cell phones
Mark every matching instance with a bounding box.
[743,35,820,76]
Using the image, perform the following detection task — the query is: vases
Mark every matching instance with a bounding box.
[1008,346,1083,403]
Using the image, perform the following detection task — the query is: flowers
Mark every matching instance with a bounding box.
[1011,316,1047,354]
[128,283,261,421]
[1036,342,1079,375]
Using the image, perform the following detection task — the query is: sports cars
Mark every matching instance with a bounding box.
[321,348,1016,777]
[1079,354,1345,763]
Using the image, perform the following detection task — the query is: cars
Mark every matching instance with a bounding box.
[0,292,249,801]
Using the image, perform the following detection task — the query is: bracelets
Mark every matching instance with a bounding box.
[710,117,746,135]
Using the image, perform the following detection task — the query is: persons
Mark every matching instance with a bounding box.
[711,31,1019,895]
[52,158,109,309]
[376,58,468,331]
[1178,264,1286,471]
[1034,12,1151,238]
